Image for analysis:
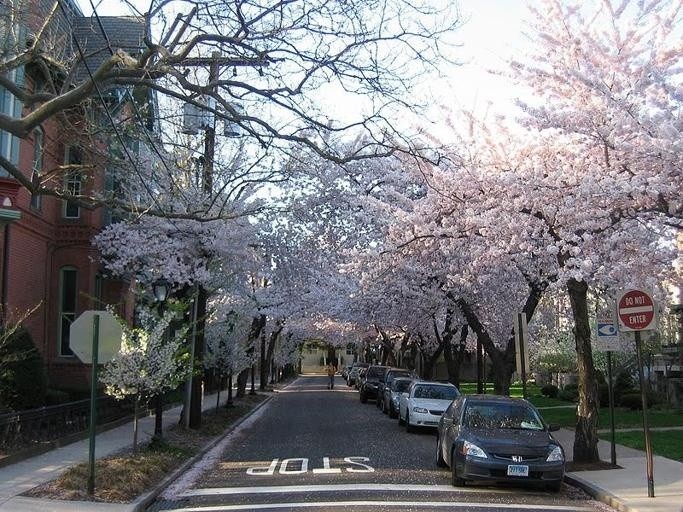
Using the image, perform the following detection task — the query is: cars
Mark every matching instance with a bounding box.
[339,362,462,433]
[435,394,565,495]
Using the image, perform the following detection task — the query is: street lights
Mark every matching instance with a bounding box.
[175,102,247,429]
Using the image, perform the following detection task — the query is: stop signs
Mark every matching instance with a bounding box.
[616,289,656,329]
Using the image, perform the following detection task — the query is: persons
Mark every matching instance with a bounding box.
[324,361,336,389]
